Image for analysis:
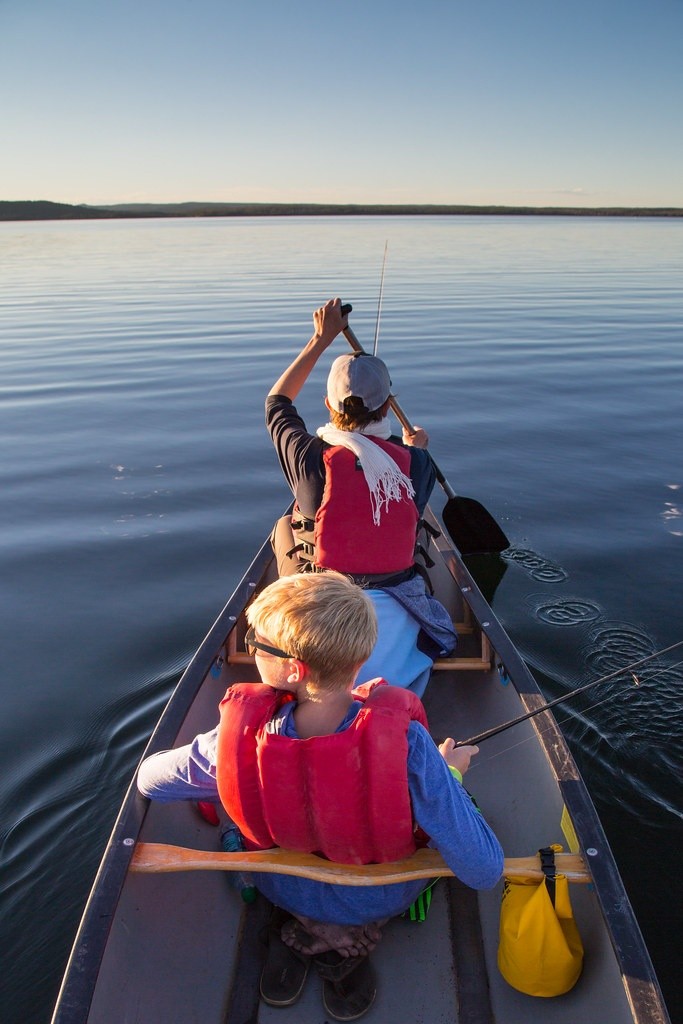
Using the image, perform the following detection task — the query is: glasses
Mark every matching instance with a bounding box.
[244,626,303,662]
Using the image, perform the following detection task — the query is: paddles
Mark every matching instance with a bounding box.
[338,301,511,557]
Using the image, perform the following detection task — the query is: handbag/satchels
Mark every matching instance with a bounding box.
[498,843,584,998]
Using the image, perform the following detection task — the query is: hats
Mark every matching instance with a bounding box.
[328,351,391,414]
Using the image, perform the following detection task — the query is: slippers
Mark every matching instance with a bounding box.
[259,906,313,1005]
[320,950,377,1021]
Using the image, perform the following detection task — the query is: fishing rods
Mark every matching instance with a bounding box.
[451,640,683,753]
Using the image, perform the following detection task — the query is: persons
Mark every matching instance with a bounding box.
[264,298,458,700]
[137,572,504,956]
